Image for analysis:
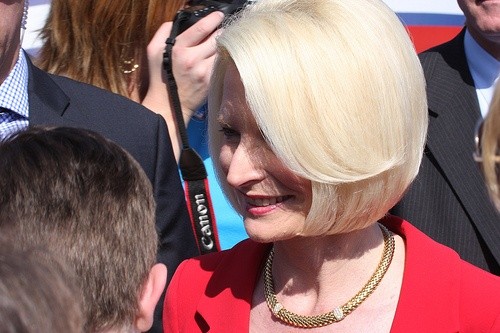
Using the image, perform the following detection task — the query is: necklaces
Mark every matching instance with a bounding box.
[262,222,396,328]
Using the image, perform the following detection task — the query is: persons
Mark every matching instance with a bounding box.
[162,0,500,333]
[385,0,500,278]
[0,0,199,333]
[32,0,250,255]
[1,122,167,333]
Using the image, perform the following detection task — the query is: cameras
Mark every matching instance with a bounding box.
[178,0,252,35]
